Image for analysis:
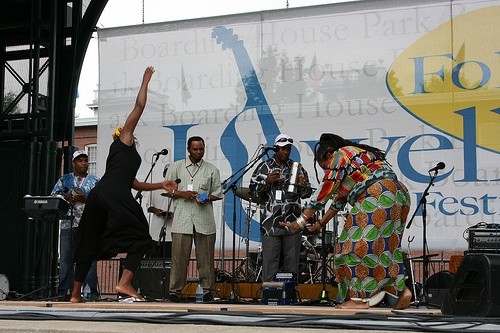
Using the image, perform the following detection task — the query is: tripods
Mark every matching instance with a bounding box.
[109,155,159,302]
[235,198,261,283]
[300,210,341,307]
[14,196,70,301]
[410,169,441,310]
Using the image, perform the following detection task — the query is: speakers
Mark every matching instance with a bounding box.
[119,258,171,299]
[441,255,500,317]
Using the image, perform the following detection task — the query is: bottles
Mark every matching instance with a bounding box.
[83,282,91,300]
[195,283,204,302]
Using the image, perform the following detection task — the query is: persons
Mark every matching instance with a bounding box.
[146,162,174,258]
[279,133,412,310]
[165,136,224,301]
[249,133,312,283]
[68,65,178,303]
[50,150,102,301]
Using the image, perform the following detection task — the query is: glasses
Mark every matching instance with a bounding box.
[275,137,294,143]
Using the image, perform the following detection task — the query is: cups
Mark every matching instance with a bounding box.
[71,186,83,198]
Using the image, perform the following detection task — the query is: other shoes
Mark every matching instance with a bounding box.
[89,293,100,301]
[203,293,215,304]
[57,294,68,301]
[166,294,180,303]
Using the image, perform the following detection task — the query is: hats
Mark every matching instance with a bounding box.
[72,149,89,162]
[273,133,295,147]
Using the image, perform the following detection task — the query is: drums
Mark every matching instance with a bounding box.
[300,206,321,234]
[255,235,319,282]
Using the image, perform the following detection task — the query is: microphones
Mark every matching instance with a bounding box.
[153,149,168,156]
[262,145,280,152]
[429,162,445,171]
[171,179,181,198]
[53,187,69,194]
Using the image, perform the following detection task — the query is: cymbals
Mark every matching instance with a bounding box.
[232,184,262,203]
[288,182,318,192]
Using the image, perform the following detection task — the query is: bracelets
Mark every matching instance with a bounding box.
[319,222,324,227]
[296,215,308,230]
[302,213,309,219]
